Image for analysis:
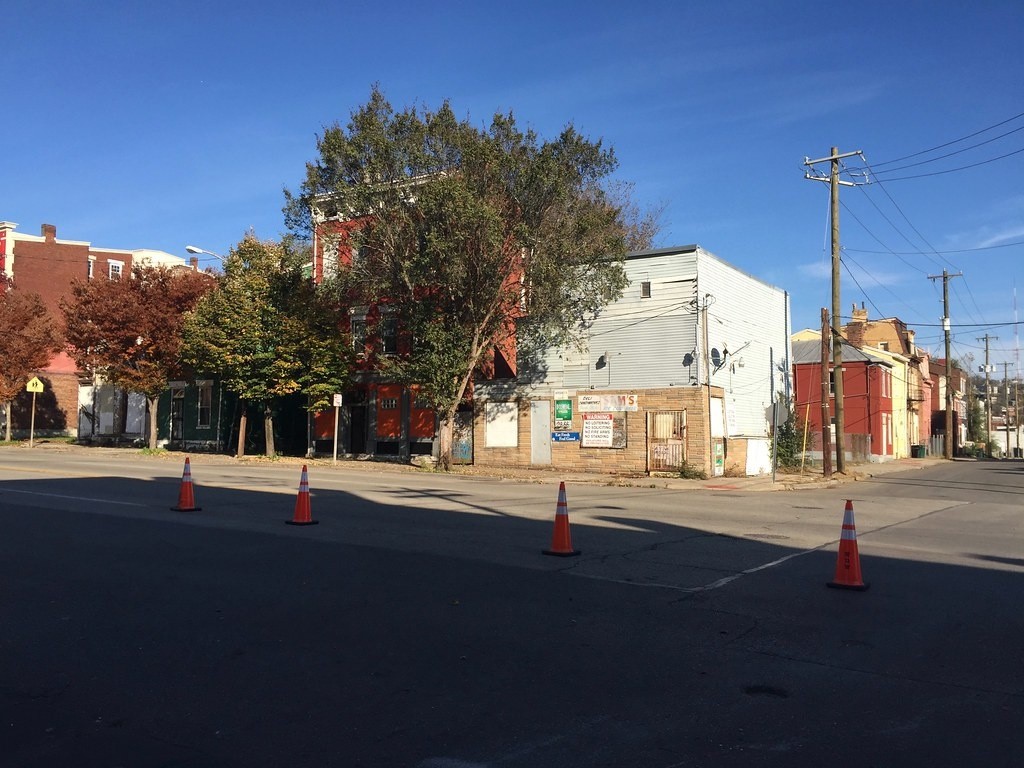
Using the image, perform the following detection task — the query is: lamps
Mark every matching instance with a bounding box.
[603,351,611,364]
[689,346,700,361]
[732,357,745,367]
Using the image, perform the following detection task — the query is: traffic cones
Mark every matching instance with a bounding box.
[541,480,581,558]
[827,499,872,592]
[284,466,320,525]
[168,458,202,512]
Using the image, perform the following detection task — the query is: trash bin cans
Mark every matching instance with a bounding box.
[911,445,925,458]
[976,448,984,458]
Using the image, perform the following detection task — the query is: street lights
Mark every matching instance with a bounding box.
[185,245,227,264]
